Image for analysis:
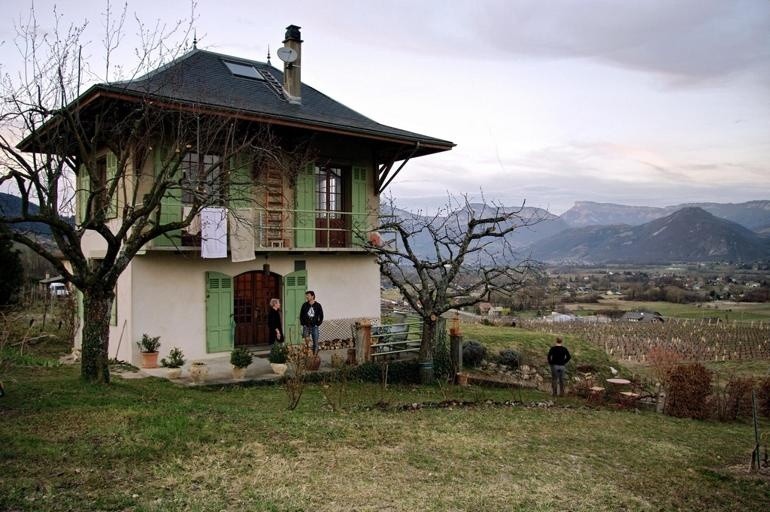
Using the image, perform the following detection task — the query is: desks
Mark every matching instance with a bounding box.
[604,377,631,413]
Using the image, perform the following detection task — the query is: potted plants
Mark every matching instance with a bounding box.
[186,359,209,384]
[228,345,253,381]
[159,346,184,379]
[134,331,160,370]
[266,338,290,375]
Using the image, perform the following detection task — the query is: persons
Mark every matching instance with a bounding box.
[547,336,572,397]
[267,298,284,345]
[299,290,324,354]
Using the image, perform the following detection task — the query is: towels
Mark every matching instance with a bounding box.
[200,207,228,258]
[229,207,256,263]
[181,207,201,235]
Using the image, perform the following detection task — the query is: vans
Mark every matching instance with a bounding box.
[49,282,69,297]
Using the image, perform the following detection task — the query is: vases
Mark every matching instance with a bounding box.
[306,357,320,370]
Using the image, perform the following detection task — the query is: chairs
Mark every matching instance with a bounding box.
[617,382,645,413]
[583,371,606,408]
